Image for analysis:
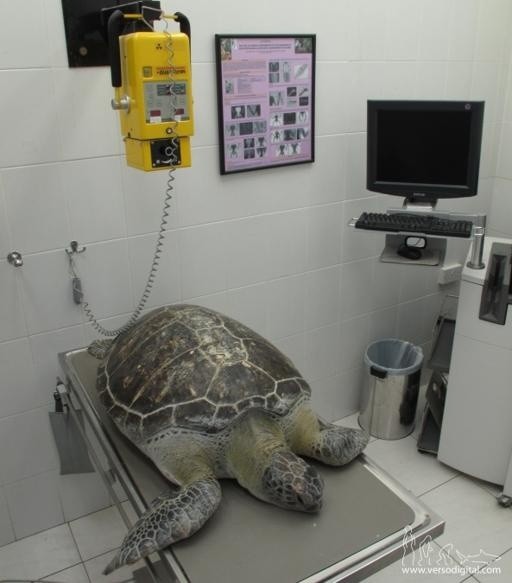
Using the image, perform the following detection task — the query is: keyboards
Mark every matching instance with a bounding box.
[354,211,474,239]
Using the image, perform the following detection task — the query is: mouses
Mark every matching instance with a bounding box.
[397,246,422,259]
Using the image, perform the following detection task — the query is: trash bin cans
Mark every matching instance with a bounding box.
[357,338,424,441]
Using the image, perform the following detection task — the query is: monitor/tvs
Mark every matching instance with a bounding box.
[365,99,485,208]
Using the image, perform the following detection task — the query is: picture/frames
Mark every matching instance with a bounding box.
[214,32,316,175]
